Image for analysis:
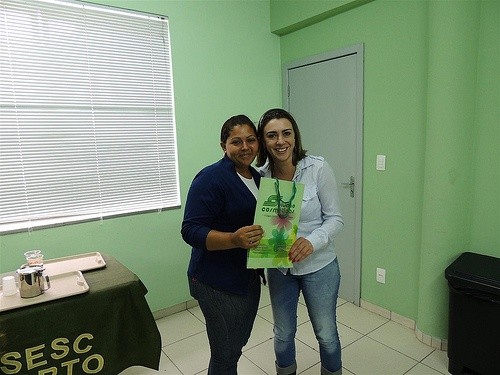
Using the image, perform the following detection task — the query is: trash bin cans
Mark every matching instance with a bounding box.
[443,252,500,375]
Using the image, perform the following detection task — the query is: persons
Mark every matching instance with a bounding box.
[257,108,344,375]
[180,115,266,375]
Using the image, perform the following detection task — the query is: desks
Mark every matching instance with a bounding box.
[444,250,500,375]
[0,252,162,375]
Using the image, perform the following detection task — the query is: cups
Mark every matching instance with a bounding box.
[2,276,17,297]
[25,250,41,260]
[27,254,43,269]
[16,268,50,297]
